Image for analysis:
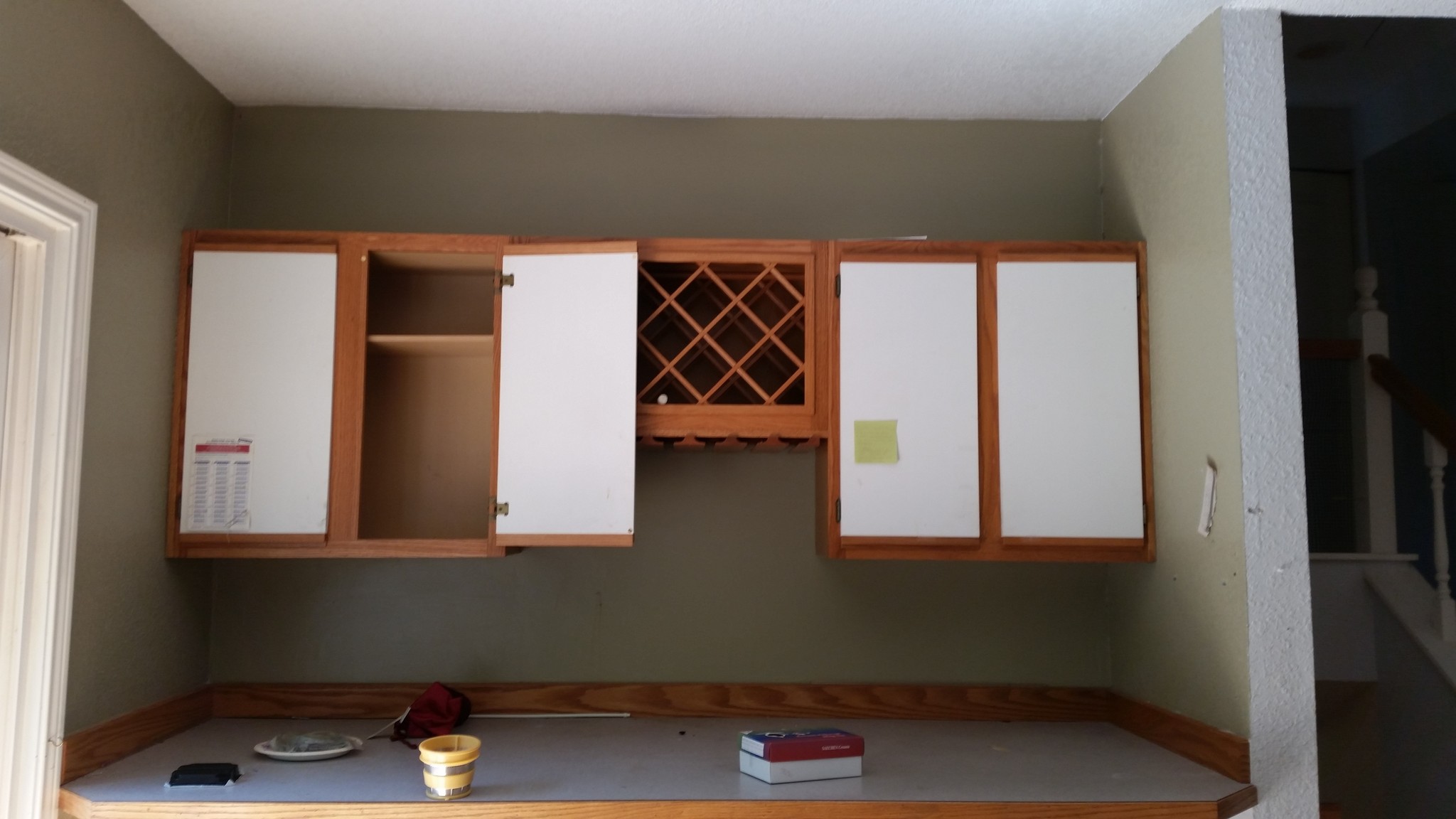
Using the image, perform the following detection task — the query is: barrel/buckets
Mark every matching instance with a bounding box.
[418,734,481,801]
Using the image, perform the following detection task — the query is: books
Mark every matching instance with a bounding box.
[740,747,864,785]
[740,727,865,762]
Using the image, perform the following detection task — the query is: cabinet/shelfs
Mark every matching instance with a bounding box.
[172,223,639,568]
[813,240,1165,567]
[638,239,823,452]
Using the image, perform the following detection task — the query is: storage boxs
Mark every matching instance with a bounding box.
[738,749,863,784]
[738,729,862,758]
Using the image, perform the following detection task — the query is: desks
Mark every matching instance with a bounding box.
[61,724,1257,819]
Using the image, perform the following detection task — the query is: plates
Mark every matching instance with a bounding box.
[253,736,364,761]
